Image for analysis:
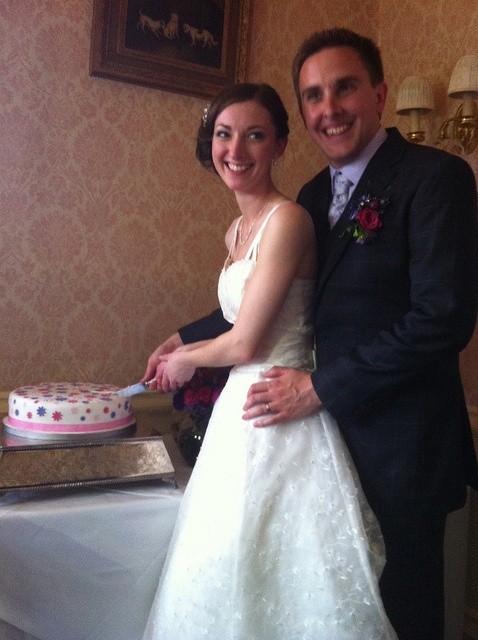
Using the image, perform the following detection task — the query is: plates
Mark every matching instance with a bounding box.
[3,416,139,438]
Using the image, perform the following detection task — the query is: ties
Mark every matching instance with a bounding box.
[327,173,353,234]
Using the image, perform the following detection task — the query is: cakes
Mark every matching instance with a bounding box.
[8,382,132,433]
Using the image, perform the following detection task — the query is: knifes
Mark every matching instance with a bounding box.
[114,378,155,400]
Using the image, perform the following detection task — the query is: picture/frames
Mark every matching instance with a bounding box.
[89,1,251,98]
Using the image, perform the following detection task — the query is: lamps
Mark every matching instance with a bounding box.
[395,75,434,144]
[447,55,478,155]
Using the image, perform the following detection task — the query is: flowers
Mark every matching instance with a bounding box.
[171,368,228,432]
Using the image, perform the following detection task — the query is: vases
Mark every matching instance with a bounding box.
[177,429,204,466]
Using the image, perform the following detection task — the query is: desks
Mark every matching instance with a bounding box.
[0,481,186,639]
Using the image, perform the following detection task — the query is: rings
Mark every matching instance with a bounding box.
[264,400,271,412]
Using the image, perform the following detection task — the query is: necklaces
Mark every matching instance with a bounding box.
[238,204,267,246]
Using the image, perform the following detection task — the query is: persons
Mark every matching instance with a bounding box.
[144,83,402,640]
[141,29,477,639]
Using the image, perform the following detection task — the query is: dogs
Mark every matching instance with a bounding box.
[182,22,219,49]
[136,8,166,39]
[161,12,180,41]
